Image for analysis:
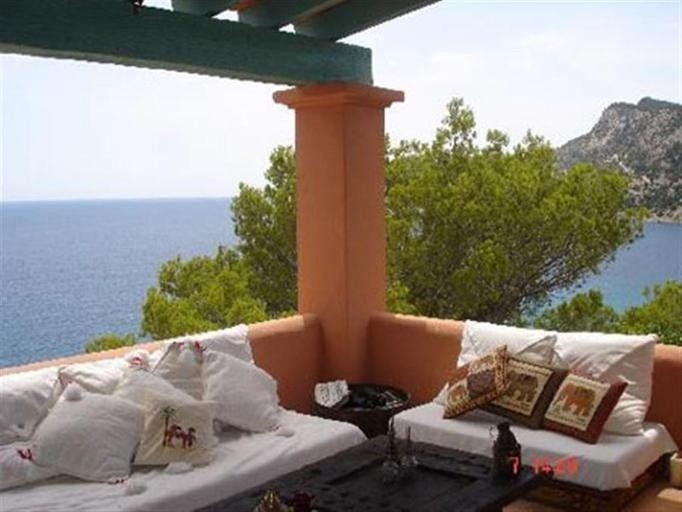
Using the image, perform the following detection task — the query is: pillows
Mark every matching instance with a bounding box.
[478,358,570,430]
[550,331,656,434]
[202,348,278,432]
[18,382,145,484]
[162,323,254,364]
[542,365,627,443]
[0,367,62,490]
[56,350,150,393]
[434,318,558,420]
[136,386,217,467]
[443,343,506,418]
[150,341,208,401]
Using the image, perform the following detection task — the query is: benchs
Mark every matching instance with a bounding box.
[0,408,369,511]
[388,402,679,511]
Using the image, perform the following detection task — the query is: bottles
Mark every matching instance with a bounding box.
[491,422,521,486]
[379,431,399,485]
[400,426,421,489]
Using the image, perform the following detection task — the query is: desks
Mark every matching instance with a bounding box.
[187,436,554,511]
